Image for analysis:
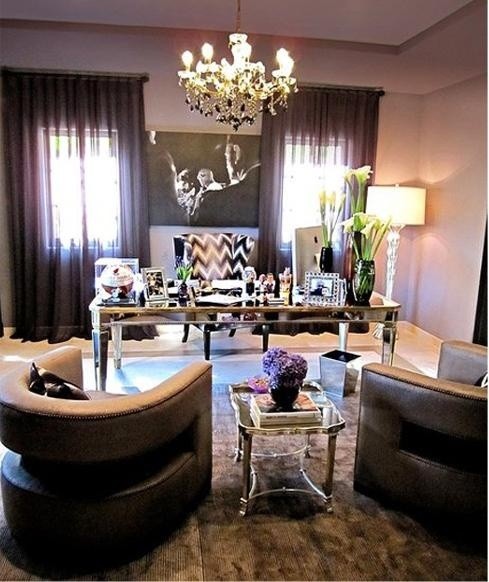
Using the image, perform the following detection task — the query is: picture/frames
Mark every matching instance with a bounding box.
[141,267,170,301]
[304,270,340,307]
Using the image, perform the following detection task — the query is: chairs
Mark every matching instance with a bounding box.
[353,340,488,557]
[171,233,269,361]
[0,346,214,573]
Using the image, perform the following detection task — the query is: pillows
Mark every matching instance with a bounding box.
[474,370,488,387]
[28,361,90,401]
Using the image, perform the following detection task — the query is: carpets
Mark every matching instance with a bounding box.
[0,346,488,582]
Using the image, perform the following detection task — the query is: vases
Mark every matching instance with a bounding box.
[269,384,299,412]
[320,245,334,274]
[352,258,375,304]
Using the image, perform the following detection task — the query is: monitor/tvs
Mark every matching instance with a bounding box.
[292,225,325,287]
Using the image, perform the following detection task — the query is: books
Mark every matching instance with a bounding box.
[249,391,322,427]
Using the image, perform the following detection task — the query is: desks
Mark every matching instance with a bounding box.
[89,288,400,388]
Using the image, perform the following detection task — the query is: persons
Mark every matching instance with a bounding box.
[310,281,332,297]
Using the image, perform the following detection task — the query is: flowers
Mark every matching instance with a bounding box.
[261,347,308,394]
[319,187,346,247]
[341,165,394,296]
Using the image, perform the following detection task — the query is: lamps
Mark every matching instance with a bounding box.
[176,0,299,132]
[365,181,427,339]
